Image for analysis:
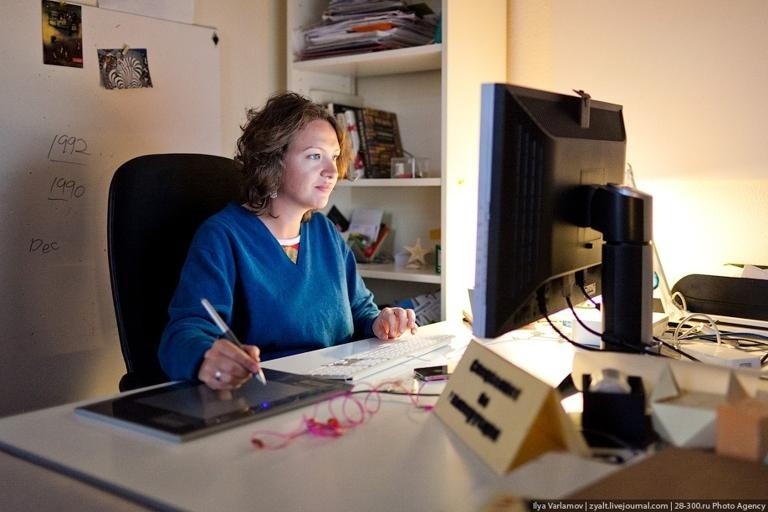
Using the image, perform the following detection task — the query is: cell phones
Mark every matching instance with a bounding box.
[414,364,456,382]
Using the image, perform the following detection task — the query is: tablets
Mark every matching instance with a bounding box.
[74,368,354,444]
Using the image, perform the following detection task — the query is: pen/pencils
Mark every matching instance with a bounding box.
[200,296,267,386]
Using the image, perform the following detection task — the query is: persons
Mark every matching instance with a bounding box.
[156,91,419,389]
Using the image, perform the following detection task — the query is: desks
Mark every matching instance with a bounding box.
[2,322,768,512]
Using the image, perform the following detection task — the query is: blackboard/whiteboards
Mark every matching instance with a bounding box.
[0,0,224,418]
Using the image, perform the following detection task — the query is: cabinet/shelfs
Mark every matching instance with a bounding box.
[284,1,509,322]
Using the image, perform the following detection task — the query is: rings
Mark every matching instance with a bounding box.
[215,369,222,381]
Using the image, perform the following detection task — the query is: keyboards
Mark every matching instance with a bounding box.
[301,334,456,382]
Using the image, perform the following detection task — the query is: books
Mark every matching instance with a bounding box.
[314,100,404,179]
[294,0,439,61]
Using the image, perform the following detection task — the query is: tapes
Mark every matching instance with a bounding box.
[588,368,632,395]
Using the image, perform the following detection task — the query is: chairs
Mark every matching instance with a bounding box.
[107,153,248,392]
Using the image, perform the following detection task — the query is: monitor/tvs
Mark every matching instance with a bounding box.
[472,83,663,356]
